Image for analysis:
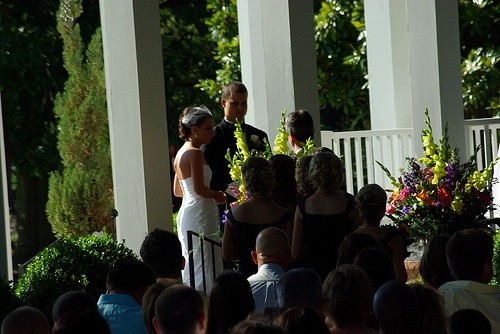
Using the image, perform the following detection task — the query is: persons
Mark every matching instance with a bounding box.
[204,82,273,233]
[222,156,295,278]
[291,152,359,278]
[0,227,500,334]
[337,184,408,283]
[173,104,226,296]
[285,110,317,157]
[268,154,318,213]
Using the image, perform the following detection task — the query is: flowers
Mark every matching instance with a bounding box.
[222,107,324,227]
[372,107,500,261]
